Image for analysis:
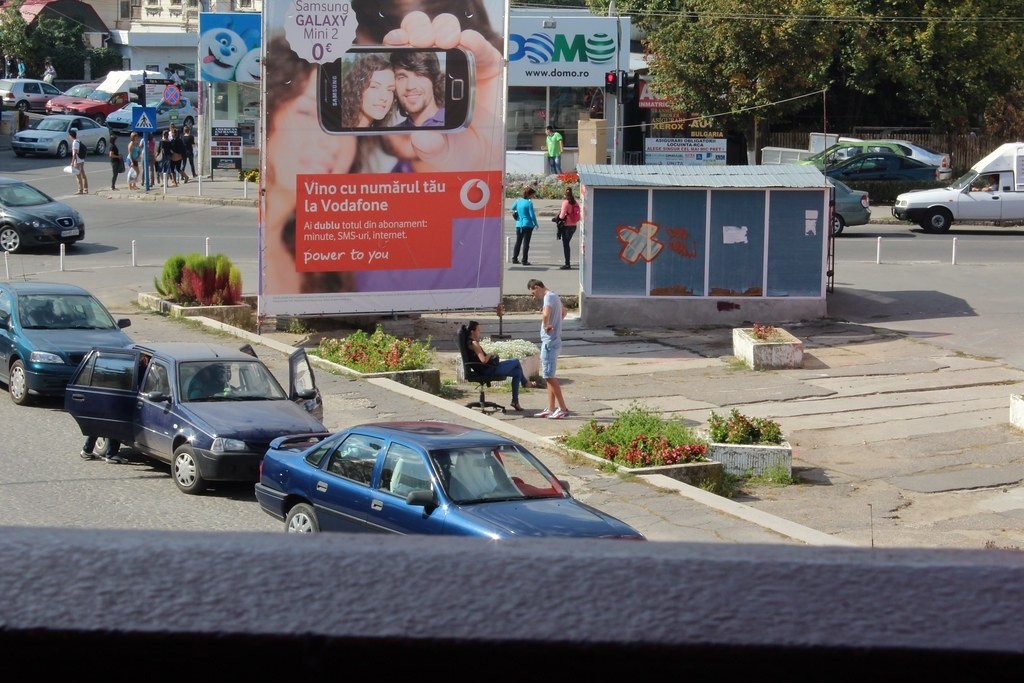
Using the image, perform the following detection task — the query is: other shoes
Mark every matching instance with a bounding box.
[74,189,83,194]
[521,259,530,265]
[560,263,570,269]
[82,188,88,194]
[512,258,521,264]
[141,173,198,186]
[129,185,140,191]
[510,402,524,410]
[522,381,538,388]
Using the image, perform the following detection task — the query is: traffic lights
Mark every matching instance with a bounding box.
[605,72,616,93]
[622,71,639,103]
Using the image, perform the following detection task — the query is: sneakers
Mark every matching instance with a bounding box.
[533,408,553,417]
[547,407,570,418]
[80,449,100,460]
[105,455,129,464]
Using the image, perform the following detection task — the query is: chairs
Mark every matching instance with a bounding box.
[458,324,507,415]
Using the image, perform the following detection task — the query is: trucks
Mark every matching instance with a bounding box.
[62,71,166,125]
[891,141,1024,235]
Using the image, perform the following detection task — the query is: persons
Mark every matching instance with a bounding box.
[465,320,538,411]
[559,187,579,269]
[974,175,998,191]
[266,0,501,294]
[210,367,236,397]
[46,62,57,86]
[80,354,148,463]
[127,123,197,190]
[109,136,123,190]
[5,55,26,79]
[511,187,539,265]
[516,123,531,150]
[528,279,569,418]
[69,130,88,195]
[165,68,181,85]
[542,126,564,175]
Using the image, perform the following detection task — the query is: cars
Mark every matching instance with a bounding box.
[0,177,84,254]
[45,83,102,115]
[0,79,64,112]
[0,282,136,406]
[826,176,872,237]
[11,115,110,159]
[105,96,197,133]
[65,341,331,494]
[255,421,647,539]
[798,139,952,183]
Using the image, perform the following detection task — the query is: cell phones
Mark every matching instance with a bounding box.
[315,46,476,134]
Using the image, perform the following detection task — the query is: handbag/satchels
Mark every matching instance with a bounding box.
[118,159,125,173]
[127,167,138,183]
[63,165,80,176]
[172,152,182,161]
[155,149,165,161]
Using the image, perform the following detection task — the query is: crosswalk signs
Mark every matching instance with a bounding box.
[132,106,156,132]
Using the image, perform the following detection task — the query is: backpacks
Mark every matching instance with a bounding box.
[567,204,580,223]
[75,141,87,159]
